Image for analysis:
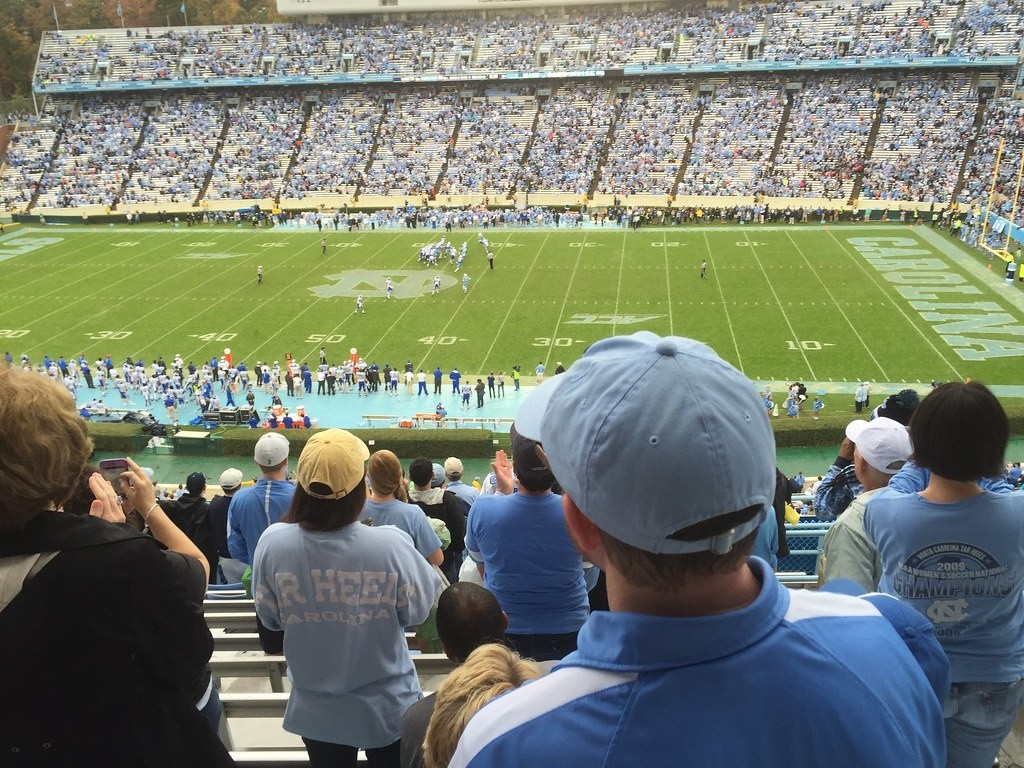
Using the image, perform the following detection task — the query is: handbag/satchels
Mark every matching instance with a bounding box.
[784,504,801,525]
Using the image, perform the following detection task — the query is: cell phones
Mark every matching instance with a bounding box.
[99,458,130,499]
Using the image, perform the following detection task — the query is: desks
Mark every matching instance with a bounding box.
[259,407,290,417]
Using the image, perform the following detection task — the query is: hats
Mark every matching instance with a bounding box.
[219,468,242,488]
[516,331,776,552]
[186,472,205,491]
[845,417,915,474]
[302,427,369,499]
[255,431,290,467]
[510,422,556,491]
[430,462,445,487]
[444,458,464,476]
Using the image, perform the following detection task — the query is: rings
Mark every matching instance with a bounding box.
[504,466,508,469]
[116,495,124,504]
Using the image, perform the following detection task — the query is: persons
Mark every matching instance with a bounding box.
[445,330,947,768]
[226,431,297,565]
[385,277,392,299]
[250,427,442,768]
[462,273,471,293]
[464,421,590,662]
[160,471,209,550]
[208,467,250,585]
[1,357,240,768]
[700,260,709,278]
[431,275,440,295]
[817,416,913,592]
[0,0,1024,768]
[321,238,327,255]
[863,380,1024,768]
[354,295,366,314]
[257,265,263,284]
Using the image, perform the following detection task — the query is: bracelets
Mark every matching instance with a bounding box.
[143,504,159,534]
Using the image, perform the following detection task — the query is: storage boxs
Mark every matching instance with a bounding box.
[203,406,256,425]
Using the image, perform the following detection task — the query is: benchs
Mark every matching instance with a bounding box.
[76,407,148,417]
[361,414,518,429]
[57,474,1024,768]
